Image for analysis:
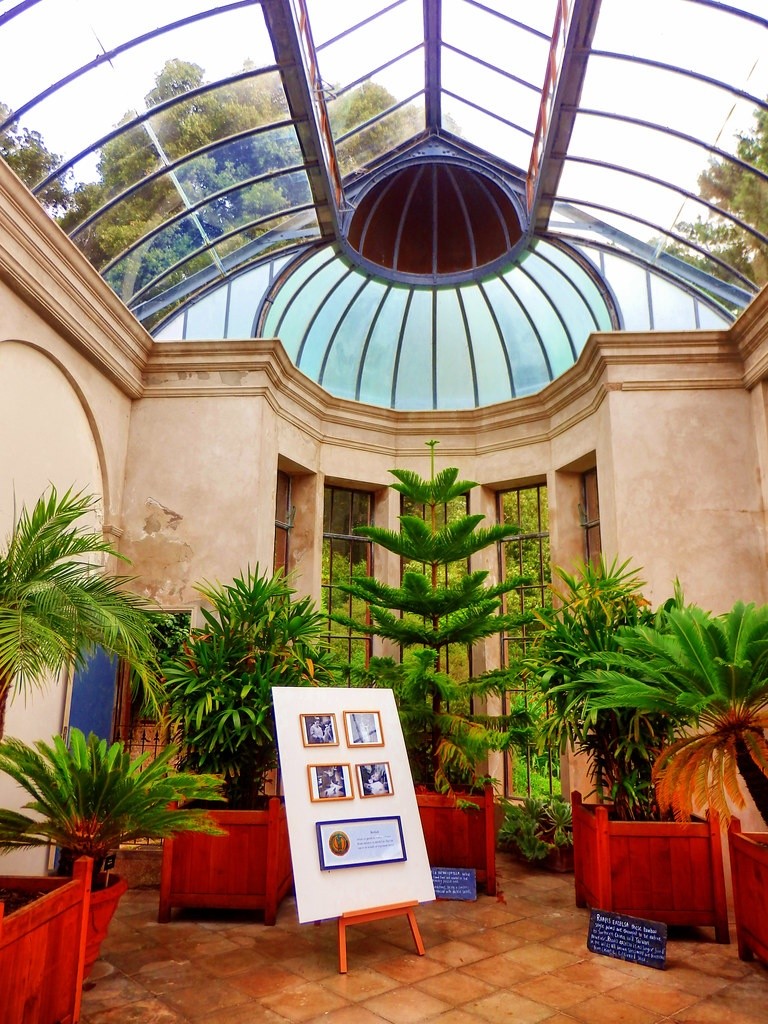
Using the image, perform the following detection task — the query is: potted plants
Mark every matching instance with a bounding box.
[515,552,731,946]
[0,475,174,1024]
[0,726,232,982]
[499,793,575,875]
[322,439,550,898]
[546,576,768,971]
[152,561,344,927]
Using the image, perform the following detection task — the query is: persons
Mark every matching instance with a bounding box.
[322,770,330,789]
[361,765,385,795]
[310,719,326,742]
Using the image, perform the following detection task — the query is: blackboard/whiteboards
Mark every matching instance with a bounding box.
[587,908,668,970]
[431,868,477,900]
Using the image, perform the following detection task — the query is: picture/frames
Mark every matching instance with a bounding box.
[356,762,395,799]
[343,710,385,749]
[299,713,340,748]
[307,763,355,803]
